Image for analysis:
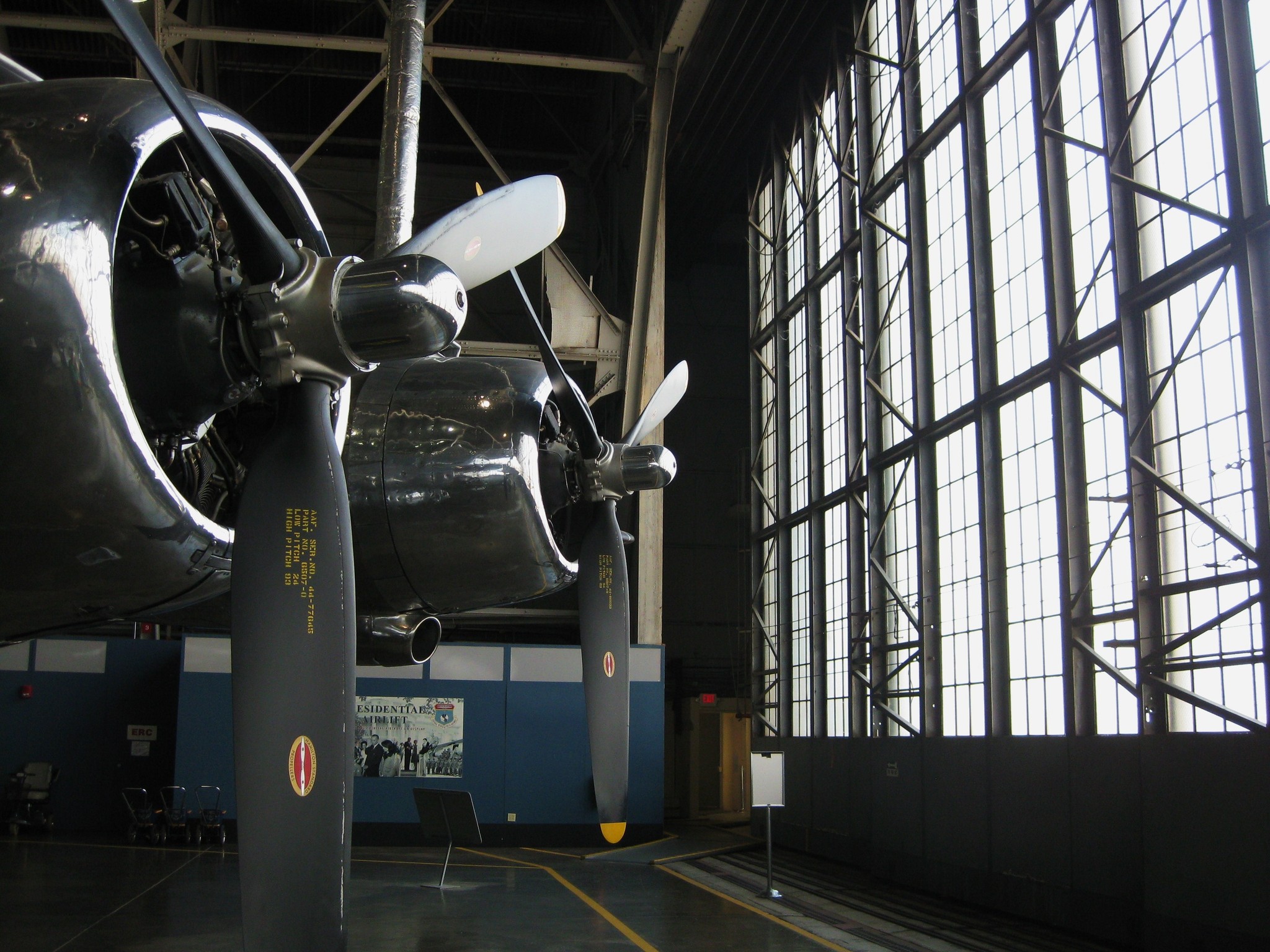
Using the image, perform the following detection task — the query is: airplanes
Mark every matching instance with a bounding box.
[1,2,691,952]
